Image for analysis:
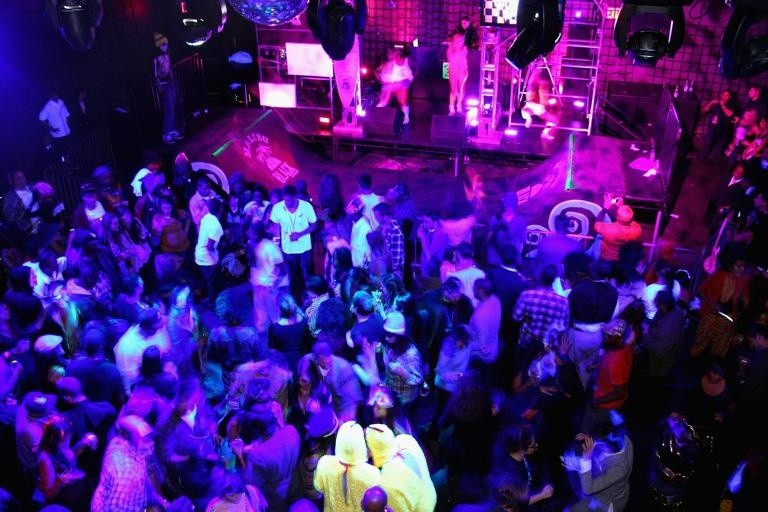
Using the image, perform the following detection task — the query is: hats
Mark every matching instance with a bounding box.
[384,312,405,336]
[25,395,49,413]
[601,319,631,337]
[35,335,63,353]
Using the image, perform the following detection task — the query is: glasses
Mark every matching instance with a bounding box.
[528,444,538,449]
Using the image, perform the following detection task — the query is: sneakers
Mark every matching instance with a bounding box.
[542,131,554,140]
[525,118,531,128]
[449,105,462,113]
[403,117,409,124]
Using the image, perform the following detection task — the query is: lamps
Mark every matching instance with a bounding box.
[57,0,104,54]
[613,0,694,65]
[306,1,368,62]
[170,1,228,48]
[504,1,565,71]
[717,0,768,80]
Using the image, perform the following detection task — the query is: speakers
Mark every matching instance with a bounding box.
[363,105,404,138]
[429,112,466,143]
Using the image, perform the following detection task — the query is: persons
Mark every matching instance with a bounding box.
[459,15,480,50]
[445,32,470,115]
[1,80,768,512]
[150,30,184,147]
[373,43,414,126]
